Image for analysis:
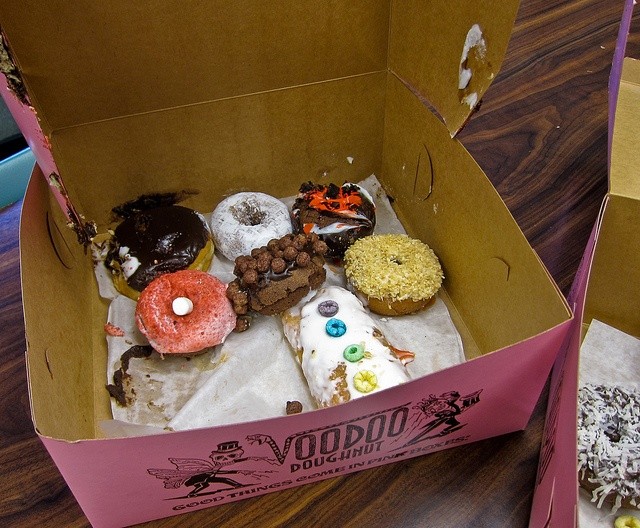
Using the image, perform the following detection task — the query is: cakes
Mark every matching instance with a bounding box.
[296,182,376,261]
[578,382,640,512]
[227,233,327,333]
[281,287,413,409]
[107,209,214,299]
[139,271,235,357]
[343,234,442,315]
[211,193,292,260]
